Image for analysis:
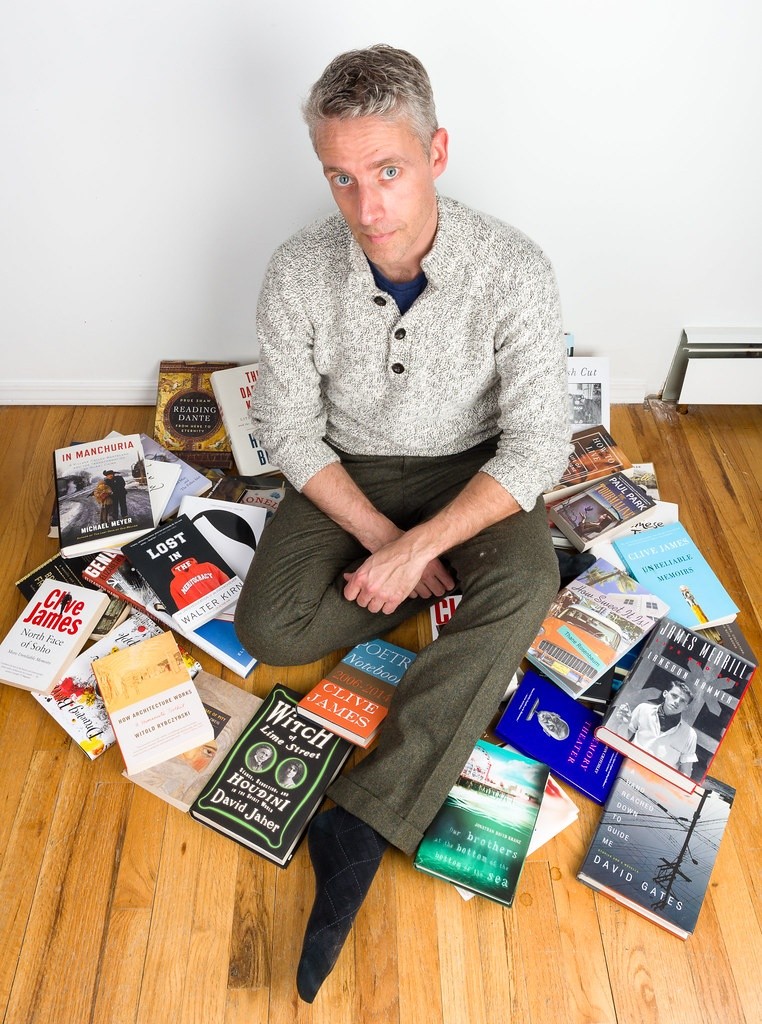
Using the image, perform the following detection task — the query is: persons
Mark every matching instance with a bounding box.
[233,45,574,1007]
[577,395,601,424]
[535,709,570,741]
[573,510,613,538]
[101,468,129,523]
[59,590,72,615]
[567,393,574,402]
[542,432,634,497]
[249,743,272,770]
[277,760,302,787]
[615,680,700,778]
[94,479,114,508]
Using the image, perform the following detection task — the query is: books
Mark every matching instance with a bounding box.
[0,320,757,943]
